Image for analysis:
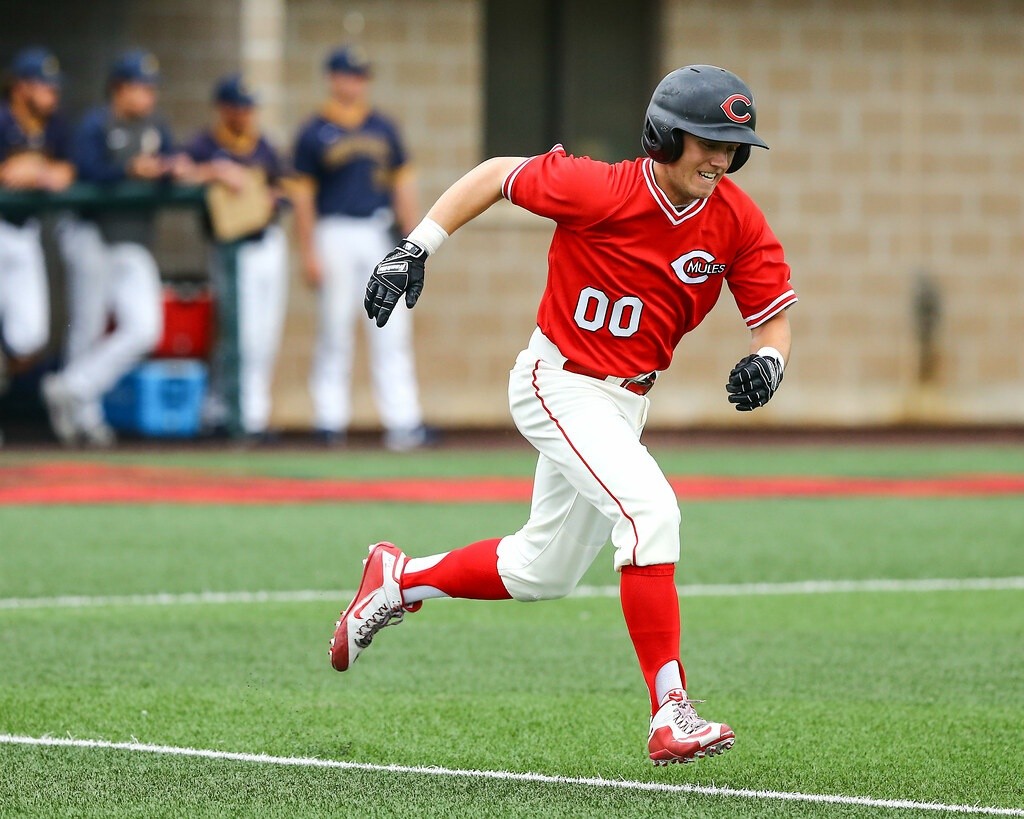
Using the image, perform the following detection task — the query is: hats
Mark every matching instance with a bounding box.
[11,55,63,85]
[209,80,261,108]
[324,45,377,78]
[118,51,175,85]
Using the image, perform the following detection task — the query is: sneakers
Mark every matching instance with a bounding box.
[41,373,119,451]
[647,698,735,765]
[329,541,423,672]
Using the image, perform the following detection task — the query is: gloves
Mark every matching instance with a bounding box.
[364,238,428,328]
[726,346,784,411]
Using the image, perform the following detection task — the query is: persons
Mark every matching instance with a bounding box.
[171,74,291,449]
[291,51,439,449]
[0,43,177,449]
[331,66,798,765]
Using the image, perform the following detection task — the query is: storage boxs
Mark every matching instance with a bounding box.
[103,279,213,358]
[103,356,207,434]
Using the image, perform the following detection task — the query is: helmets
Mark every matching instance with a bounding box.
[640,65,769,174]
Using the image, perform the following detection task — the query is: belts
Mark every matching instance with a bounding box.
[563,360,656,396]
